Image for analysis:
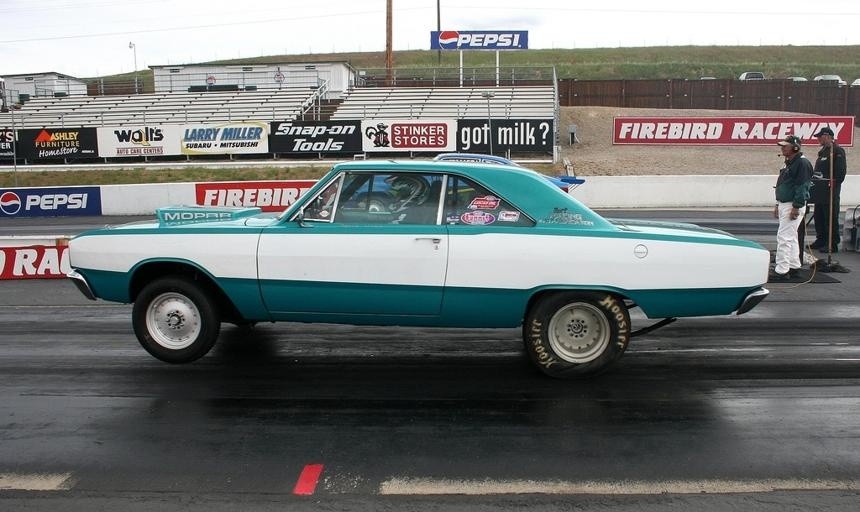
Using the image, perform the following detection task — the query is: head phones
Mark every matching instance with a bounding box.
[790,135,800,153]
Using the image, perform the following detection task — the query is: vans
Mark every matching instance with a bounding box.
[737,71,766,80]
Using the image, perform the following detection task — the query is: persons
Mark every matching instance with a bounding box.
[805,127,848,253]
[769,134,814,283]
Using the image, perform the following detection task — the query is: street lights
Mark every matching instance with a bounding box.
[128,42,138,94]
[481,90,497,155]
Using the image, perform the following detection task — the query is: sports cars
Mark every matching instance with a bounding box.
[59,152,775,385]
[341,152,590,214]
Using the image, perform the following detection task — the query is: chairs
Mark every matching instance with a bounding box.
[423,181,449,224]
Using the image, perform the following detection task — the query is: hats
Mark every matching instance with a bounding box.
[777,136,801,147]
[814,128,834,137]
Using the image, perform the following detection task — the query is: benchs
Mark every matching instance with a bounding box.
[0,89,319,129]
[329,86,555,120]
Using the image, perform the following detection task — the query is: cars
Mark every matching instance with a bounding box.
[786,76,809,82]
[814,74,848,88]
[849,77,860,88]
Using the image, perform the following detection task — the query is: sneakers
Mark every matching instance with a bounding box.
[806,238,825,249]
[820,243,838,252]
[769,266,802,280]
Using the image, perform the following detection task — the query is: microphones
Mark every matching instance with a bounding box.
[778,155,793,157]
